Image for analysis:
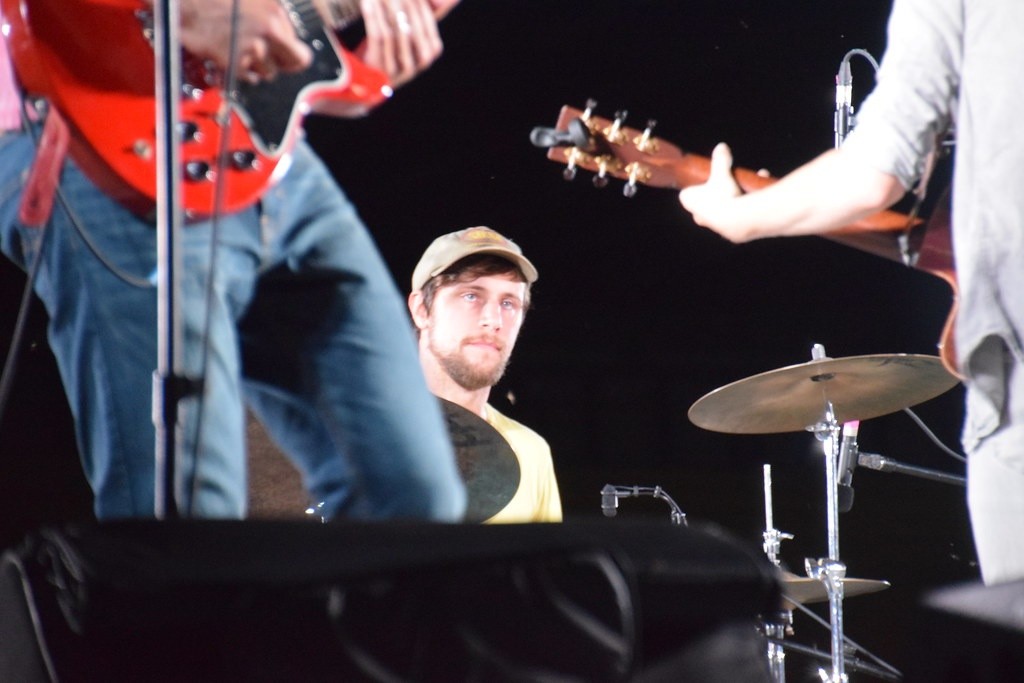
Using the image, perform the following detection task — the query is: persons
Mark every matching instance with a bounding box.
[411,226,567,527]
[0,0,470,530]
[677,0,1023,591]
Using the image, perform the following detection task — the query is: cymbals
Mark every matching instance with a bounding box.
[244,393,521,523]
[688,353,958,435]
[778,569,891,609]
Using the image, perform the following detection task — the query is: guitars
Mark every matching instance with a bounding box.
[528,100,961,380]
[3,1,460,226]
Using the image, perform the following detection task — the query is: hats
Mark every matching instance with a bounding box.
[413,226,538,293]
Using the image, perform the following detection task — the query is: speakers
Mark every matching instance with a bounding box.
[1,520,788,682]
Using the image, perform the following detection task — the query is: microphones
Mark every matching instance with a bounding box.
[601,484,618,518]
[835,420,861,512]
[832,60,857,149]
